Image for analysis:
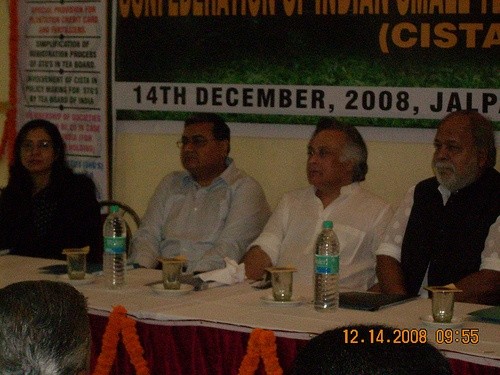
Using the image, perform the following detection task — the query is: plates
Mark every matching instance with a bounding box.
[419,314,464,328]
[59,273,95,286]
[150,281,195,296]
[260,295,305,307]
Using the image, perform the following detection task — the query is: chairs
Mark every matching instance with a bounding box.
[99,200,142,259]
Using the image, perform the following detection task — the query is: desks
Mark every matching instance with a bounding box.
[0,252,500,375]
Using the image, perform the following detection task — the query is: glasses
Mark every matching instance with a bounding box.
[180,135,216,146]
[19,139,50,150]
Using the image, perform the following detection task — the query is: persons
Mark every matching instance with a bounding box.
[0,280,92,375]
[130,112,269,275]
[285,324,454,375]
[367,110,500,305]
[243,117,393,292]
[0,120,104,263]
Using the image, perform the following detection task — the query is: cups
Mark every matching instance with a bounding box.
[265,264,297,300]
[63,246,90,278]
[423,283,463,323]
[157,257,188,289]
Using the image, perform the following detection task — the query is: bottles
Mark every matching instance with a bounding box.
[313,221,339,313]
[103,206,128,291]
